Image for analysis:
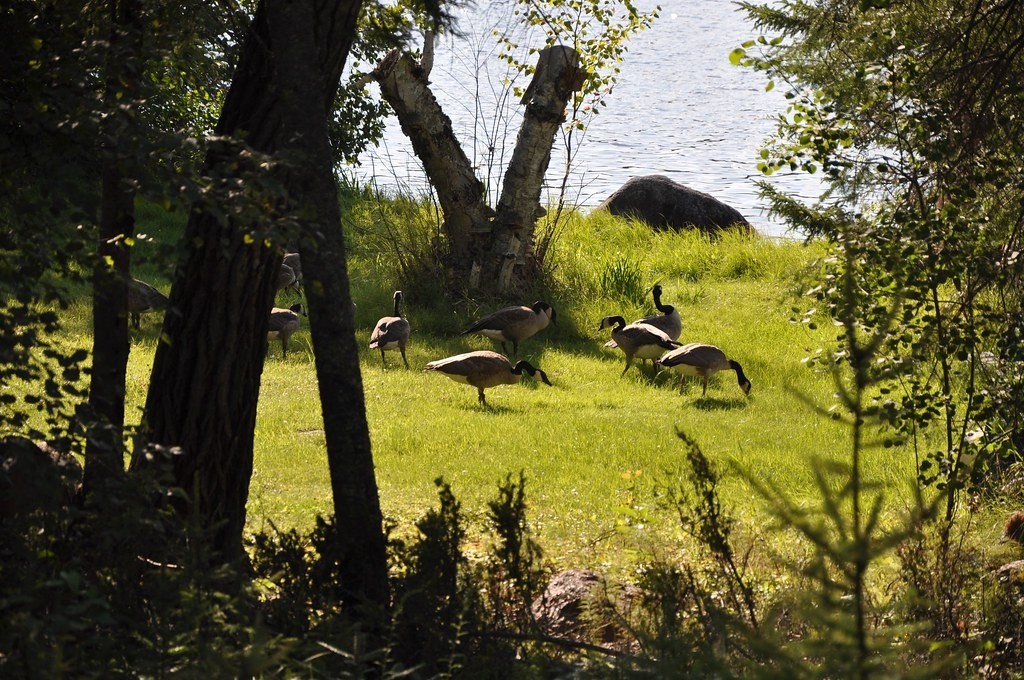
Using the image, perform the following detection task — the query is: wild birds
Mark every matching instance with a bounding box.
[598,284,752,399]
[368,290,410,370]
[267,249,307,359]
[423,351,553,407]
[459,300,558,356]
[127,273,169,328]
